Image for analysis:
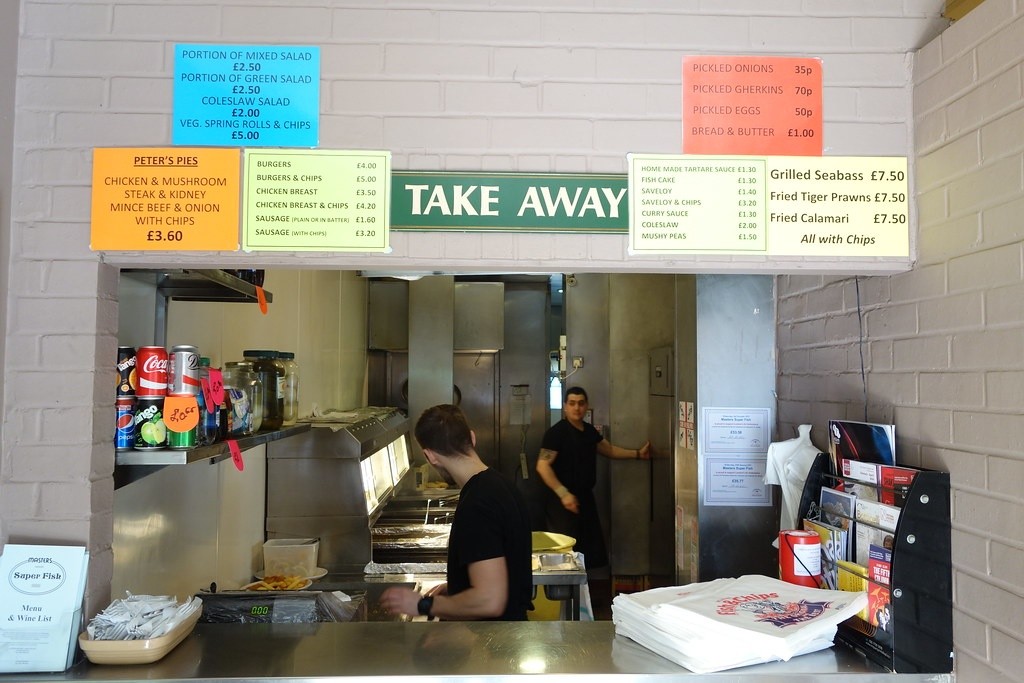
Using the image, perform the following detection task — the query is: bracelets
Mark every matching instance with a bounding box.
[556,485,568,498]
[636,448,641,460]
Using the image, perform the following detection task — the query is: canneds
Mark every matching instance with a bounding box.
[222,348,299,432]
[115,344,230,451]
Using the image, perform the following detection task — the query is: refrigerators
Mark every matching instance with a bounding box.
[646,343,675,589]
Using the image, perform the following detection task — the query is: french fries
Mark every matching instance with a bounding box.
[256,575,306,590]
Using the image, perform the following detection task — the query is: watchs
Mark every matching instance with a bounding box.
[417,596,433,616]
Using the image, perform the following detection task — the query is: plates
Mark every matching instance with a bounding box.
[254,567,328,582]
[240,578,312,591]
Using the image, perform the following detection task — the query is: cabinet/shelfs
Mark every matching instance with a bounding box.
[115,269,311,464]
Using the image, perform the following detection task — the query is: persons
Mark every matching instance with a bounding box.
[379,404,530,621]
[535,387,652,568]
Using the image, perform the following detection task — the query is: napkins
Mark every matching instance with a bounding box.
[611,574,869,674]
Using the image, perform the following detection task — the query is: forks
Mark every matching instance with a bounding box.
[86,591,202,640]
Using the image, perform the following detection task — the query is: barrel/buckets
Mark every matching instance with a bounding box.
[527,530,577,620]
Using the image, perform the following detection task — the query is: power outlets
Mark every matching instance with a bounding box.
[572,356,584,368]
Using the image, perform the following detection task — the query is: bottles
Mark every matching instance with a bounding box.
[197,357,217,446]
[277,352,300,427]
[221,361,263,434]
[240,350,286,430]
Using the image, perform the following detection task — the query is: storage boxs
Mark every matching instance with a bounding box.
[262,538,319,577]
[612,575,643,592]
[644,576,675,591]
